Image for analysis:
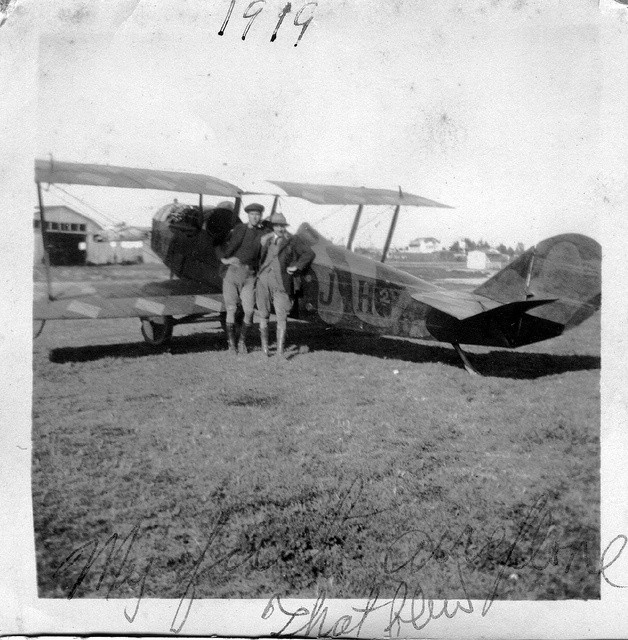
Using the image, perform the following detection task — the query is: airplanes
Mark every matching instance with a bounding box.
[136,202,601,380]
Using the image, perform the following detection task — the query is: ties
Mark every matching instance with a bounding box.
[274,235,281,245]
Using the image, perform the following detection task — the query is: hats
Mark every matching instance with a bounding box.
[245,204,264,212]
[262,212,289,226]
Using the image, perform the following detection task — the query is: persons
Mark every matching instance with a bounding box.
[255,213,316,358]
[212,203,274,356]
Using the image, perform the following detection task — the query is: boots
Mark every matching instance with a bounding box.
[237,321,252,355]
[226,322,237,357]
[276,329,286,363]
[258,328,269,357]
